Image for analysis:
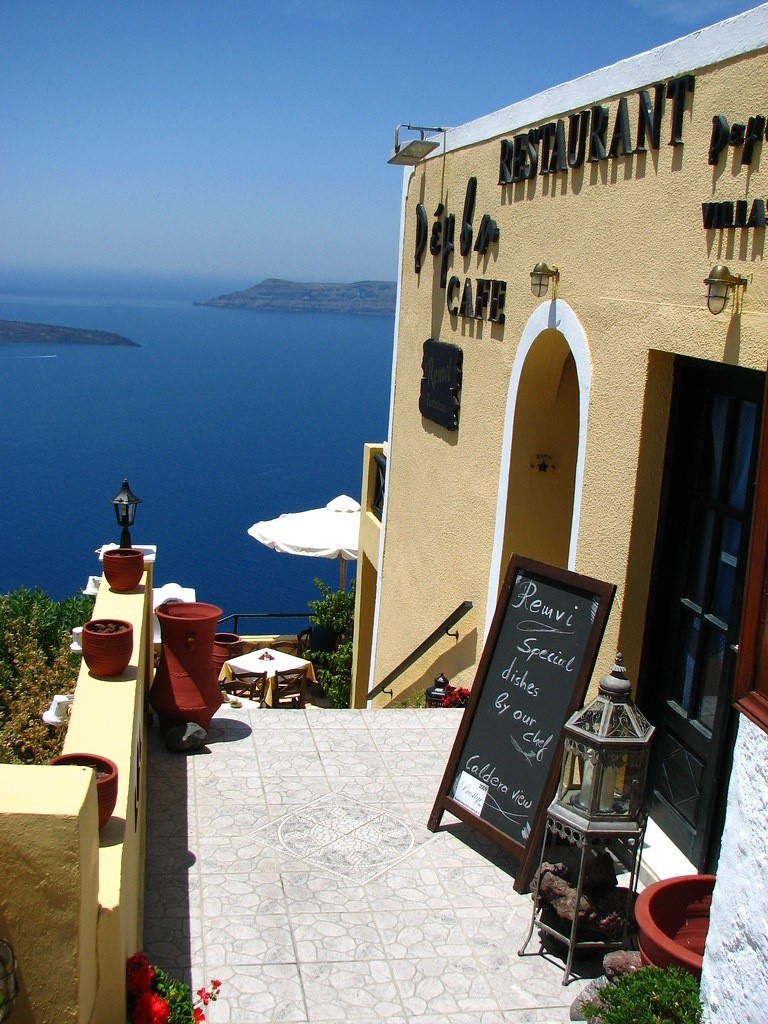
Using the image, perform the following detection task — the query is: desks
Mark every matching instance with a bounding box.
[217,647,319,709]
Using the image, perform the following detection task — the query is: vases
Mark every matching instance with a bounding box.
[212,632,244,677]
[82,619,133,678]
[145,601,225,731]
[102,549,144,592]
[46,753,118,831]
[634,874,716,983]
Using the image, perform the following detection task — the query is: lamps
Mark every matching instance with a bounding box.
[387,122,449,166]
[517,651,656,986]
[530,261,560,297]
[0,937,20,1023]
[109,479,143,549]
[703,264,747,315]
[42,542,115,726]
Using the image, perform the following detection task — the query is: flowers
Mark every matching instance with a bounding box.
[126,951,224,1024]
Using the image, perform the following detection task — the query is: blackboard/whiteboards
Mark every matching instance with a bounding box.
[441,552,617,857]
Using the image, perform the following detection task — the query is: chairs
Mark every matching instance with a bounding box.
[222,624,313,708]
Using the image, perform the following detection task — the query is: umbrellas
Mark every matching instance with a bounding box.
[246,491,363,591]
